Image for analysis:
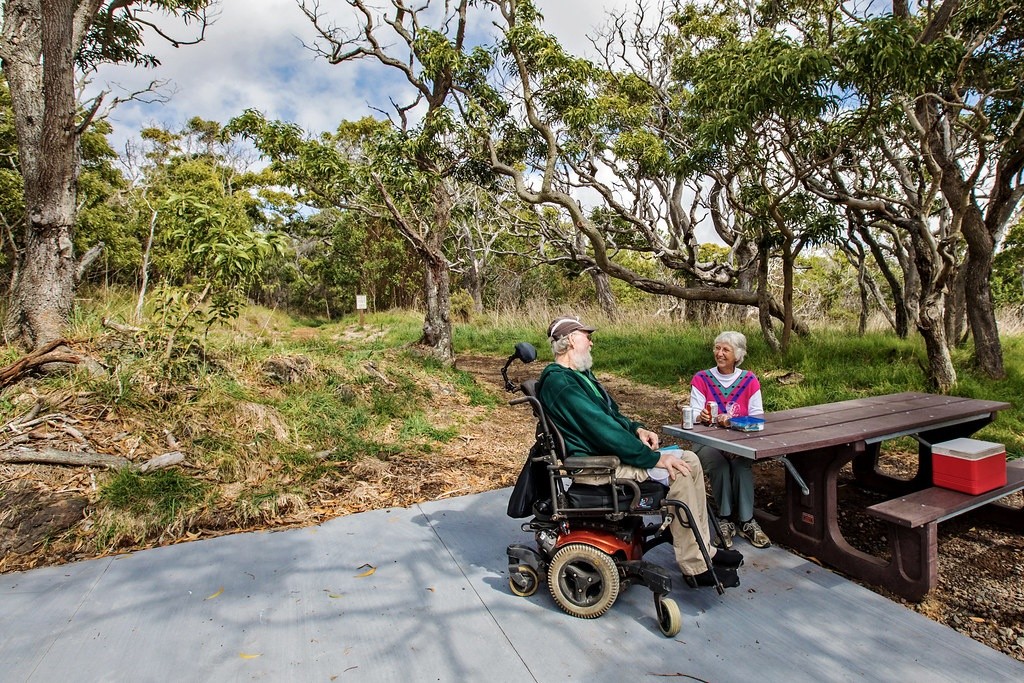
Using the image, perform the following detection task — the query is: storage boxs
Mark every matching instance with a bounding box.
[932,438,1007,495]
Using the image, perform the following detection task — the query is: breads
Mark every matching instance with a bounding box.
[716,414,735,428]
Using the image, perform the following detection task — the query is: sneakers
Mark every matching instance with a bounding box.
[736,519,772,548]
[713,521,736,547]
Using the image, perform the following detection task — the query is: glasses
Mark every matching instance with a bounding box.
[572,333,593,341]
[713,346,733,353]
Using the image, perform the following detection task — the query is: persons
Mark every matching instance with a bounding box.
[689,331,771,549]
[538,316,744,587]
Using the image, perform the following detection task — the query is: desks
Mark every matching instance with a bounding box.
[661,391,1024,603]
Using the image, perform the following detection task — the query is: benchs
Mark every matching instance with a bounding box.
[866,456,1024,603]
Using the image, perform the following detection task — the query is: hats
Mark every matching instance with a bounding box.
[547,317,599,343]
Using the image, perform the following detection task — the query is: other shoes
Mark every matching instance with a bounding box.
[683,566,737,589]
[712,549,743,569]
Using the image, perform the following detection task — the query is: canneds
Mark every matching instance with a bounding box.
[682,406,693,430]
[706,401,718,427]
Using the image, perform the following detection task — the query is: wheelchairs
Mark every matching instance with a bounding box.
[499,342,745,637]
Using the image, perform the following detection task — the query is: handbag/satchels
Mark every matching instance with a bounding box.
[507,442,550,518]
